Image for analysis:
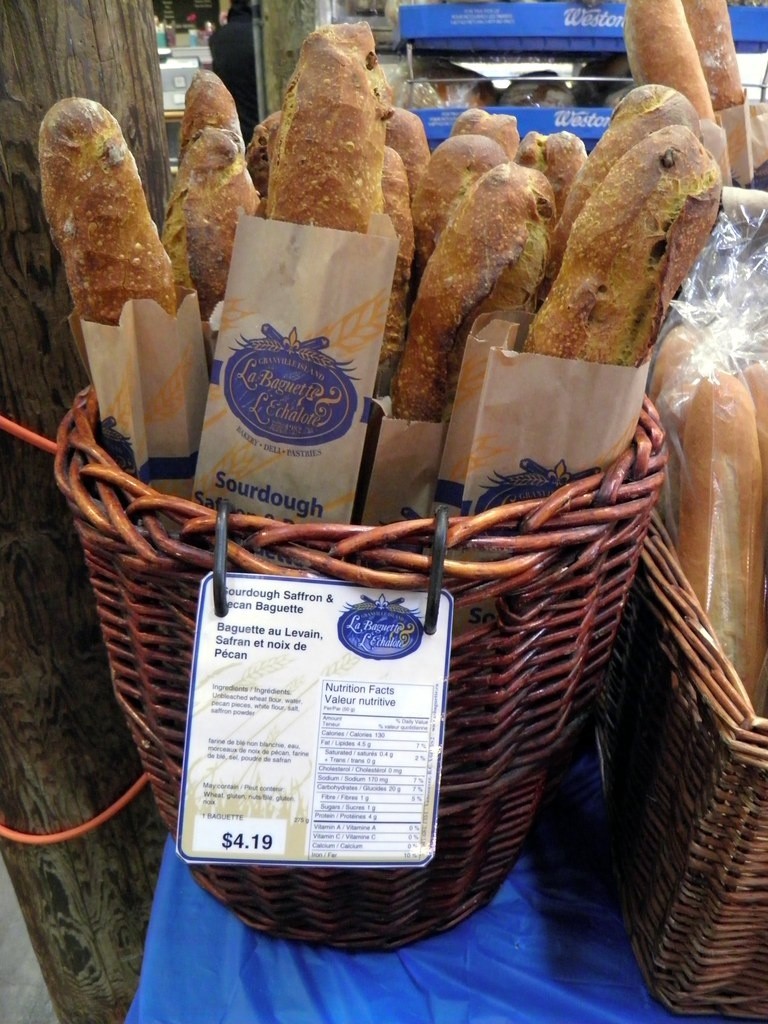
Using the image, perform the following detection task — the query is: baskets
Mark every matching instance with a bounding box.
[51,379,669,955]
[593,504,768,1024]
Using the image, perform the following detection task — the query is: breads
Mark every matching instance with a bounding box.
[36,0,768,723]
